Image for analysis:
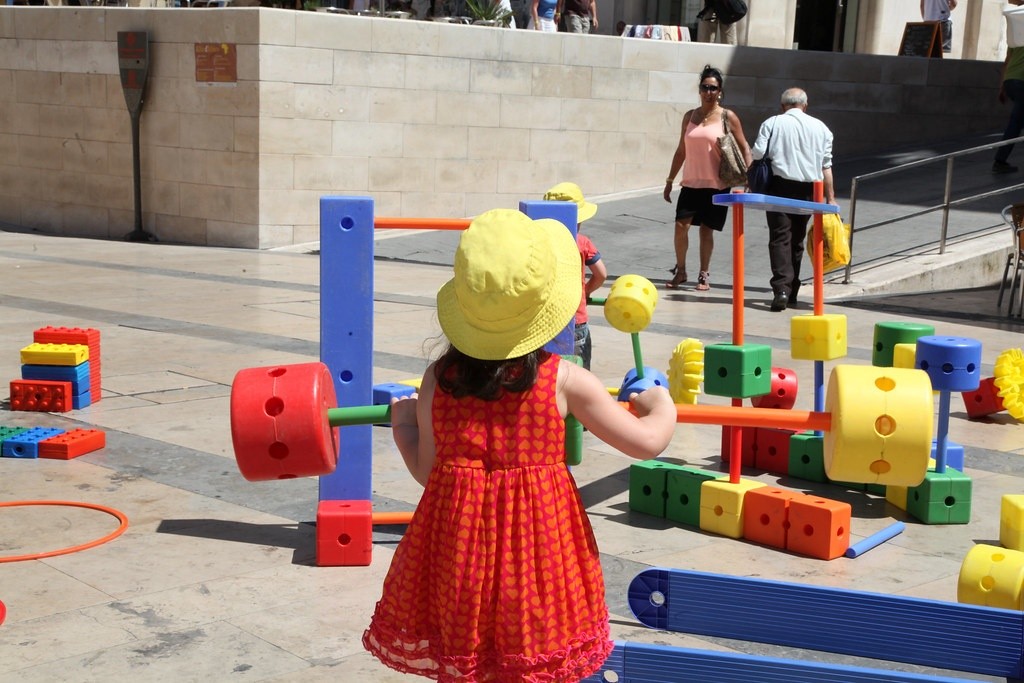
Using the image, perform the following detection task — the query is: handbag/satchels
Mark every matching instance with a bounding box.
[699,0,747,24]
[717,108,748,187]
[745,129,774,193]
[805,212,850,272]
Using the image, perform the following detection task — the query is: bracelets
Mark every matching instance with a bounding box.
[555,13,561,16]
[665,178,674,183]
[534,20,539,25]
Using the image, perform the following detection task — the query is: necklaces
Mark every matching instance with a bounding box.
[704,107,719,122]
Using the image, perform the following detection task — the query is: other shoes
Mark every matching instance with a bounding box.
[772,292,789,310]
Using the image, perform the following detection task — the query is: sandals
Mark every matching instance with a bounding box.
[698,272,710,290]
[666,269,687,289]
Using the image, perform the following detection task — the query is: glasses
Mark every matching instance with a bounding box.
[699,84,719,91]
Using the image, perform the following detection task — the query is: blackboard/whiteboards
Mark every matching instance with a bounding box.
[897,20,943,59]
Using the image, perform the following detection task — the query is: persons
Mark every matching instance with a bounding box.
[360,208,679,683]
[554,0,598,34]
[992,46,1024,174]
[542,181,606,373]
[696,0,737,46]
[919,0,956,54]
[488,0,517,29]
[749,86,838,311]
[664,64,753,290]
[527,0,559,32]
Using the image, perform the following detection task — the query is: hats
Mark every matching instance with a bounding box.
[436,208,582,360]
[544,181,597,224]
